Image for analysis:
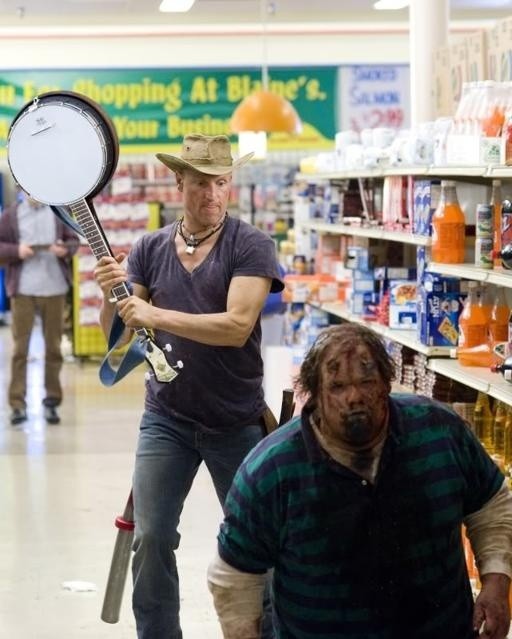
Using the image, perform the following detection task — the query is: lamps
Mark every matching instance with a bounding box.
[227,0,303,134]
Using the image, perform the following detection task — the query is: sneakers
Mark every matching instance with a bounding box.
[42,404,62,424]
[9,405,28,425]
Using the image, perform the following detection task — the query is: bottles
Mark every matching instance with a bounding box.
[472,390,512,472]
[456,80,512,170]
[432,180,466,263]
[451,282,512,381]
[491,180,502,268]
[502,195,512,271]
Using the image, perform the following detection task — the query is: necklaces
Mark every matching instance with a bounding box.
[175,219,224,255]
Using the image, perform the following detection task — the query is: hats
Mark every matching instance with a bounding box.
[154,132,255,176]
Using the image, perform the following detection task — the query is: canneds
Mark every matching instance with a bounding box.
[475,239,492,269]
[476,204,495,239]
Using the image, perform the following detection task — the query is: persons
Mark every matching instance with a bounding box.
[95,134,284,639]
[206,322,511,639]
[0,190,81,424]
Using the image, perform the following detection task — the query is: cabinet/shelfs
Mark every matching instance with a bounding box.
[105,173,185,210]
[230,165,291,236]
[70,201,159,356]
[291,163,512,406]
